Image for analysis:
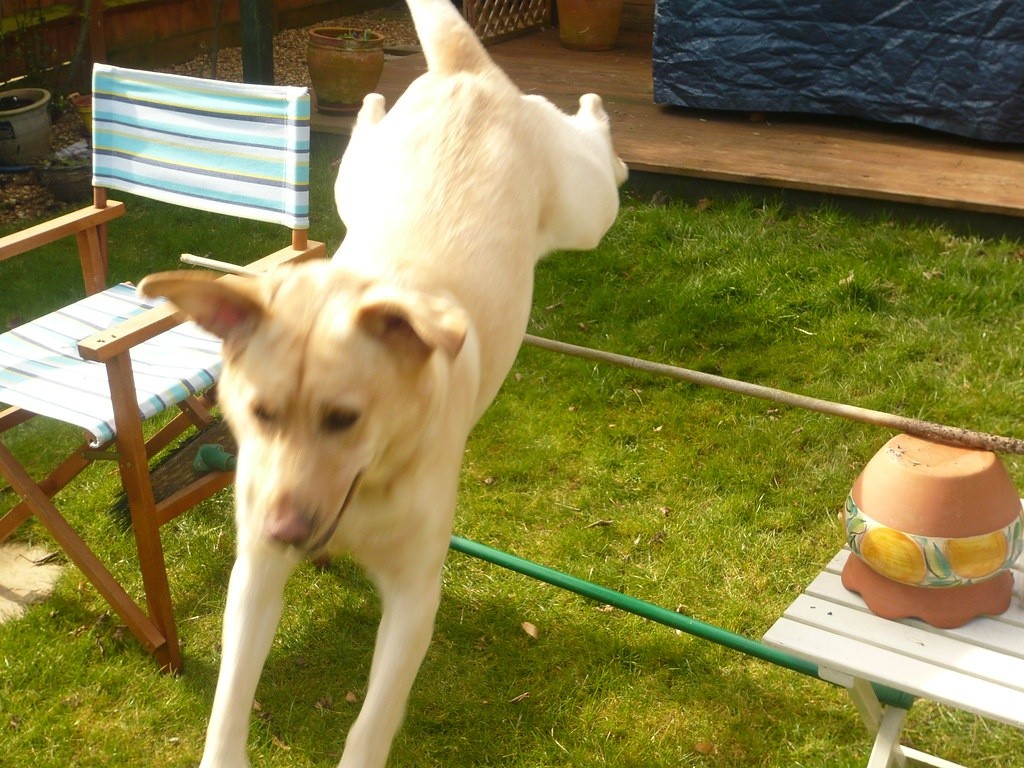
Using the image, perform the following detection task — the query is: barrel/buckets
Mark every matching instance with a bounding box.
[69,92,92,135]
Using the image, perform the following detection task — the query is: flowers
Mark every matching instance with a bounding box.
[337,28,372,42]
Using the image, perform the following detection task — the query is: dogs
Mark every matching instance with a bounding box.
[133,0,633,768]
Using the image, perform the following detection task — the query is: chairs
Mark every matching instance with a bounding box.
[0,62,325,674]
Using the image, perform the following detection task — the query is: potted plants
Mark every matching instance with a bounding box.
[34,154,93,202]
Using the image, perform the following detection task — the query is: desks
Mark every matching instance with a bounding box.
[761,497,1024,768]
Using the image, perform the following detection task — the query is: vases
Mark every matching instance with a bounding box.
[1,87,51,166]
[73,94,92,135]
[307,27,385,115]
[556,0,622,52]
